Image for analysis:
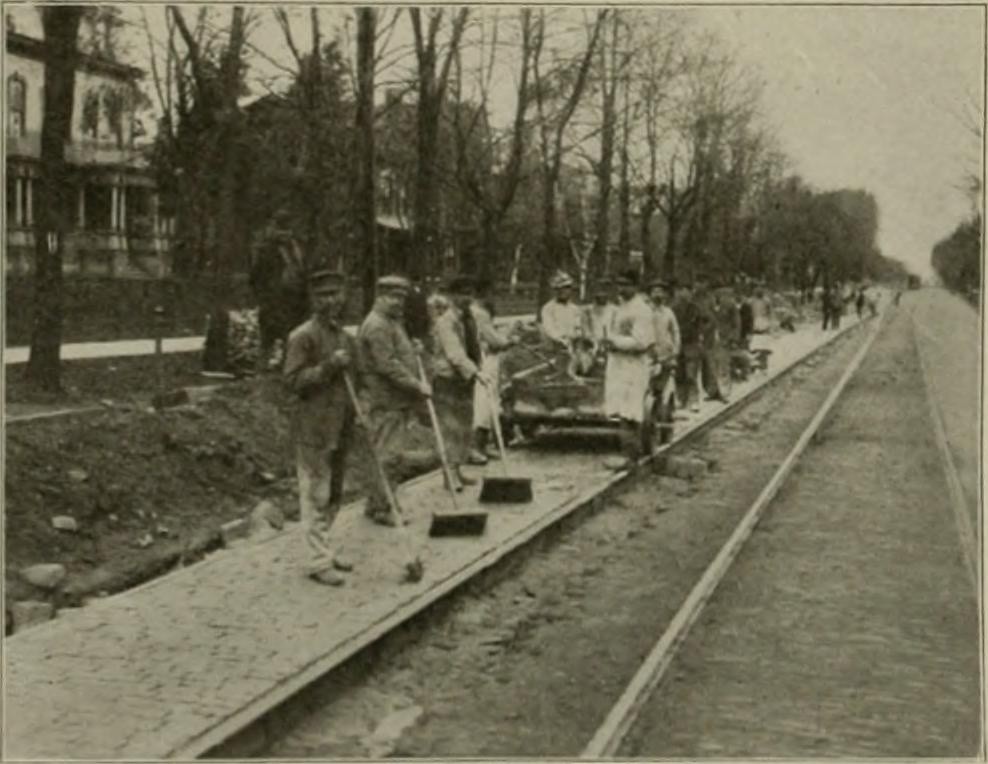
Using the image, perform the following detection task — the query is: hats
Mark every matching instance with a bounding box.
[373,276,409,297]
[648,279,671,293]
[616,268,641,285]
[309,270,345,295]
[549,269,573,288]
[449,277,474,293]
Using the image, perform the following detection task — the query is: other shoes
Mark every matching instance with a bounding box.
[332,553,353,572]
[456,465,477,485]
[312,568,344,586]
[366,507,400,518]
[482,448,499,459]
[443,470,463,491]
[375,510,408,527]
[468,450,487,465]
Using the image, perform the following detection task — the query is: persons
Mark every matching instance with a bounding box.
[282,262,903,587]
